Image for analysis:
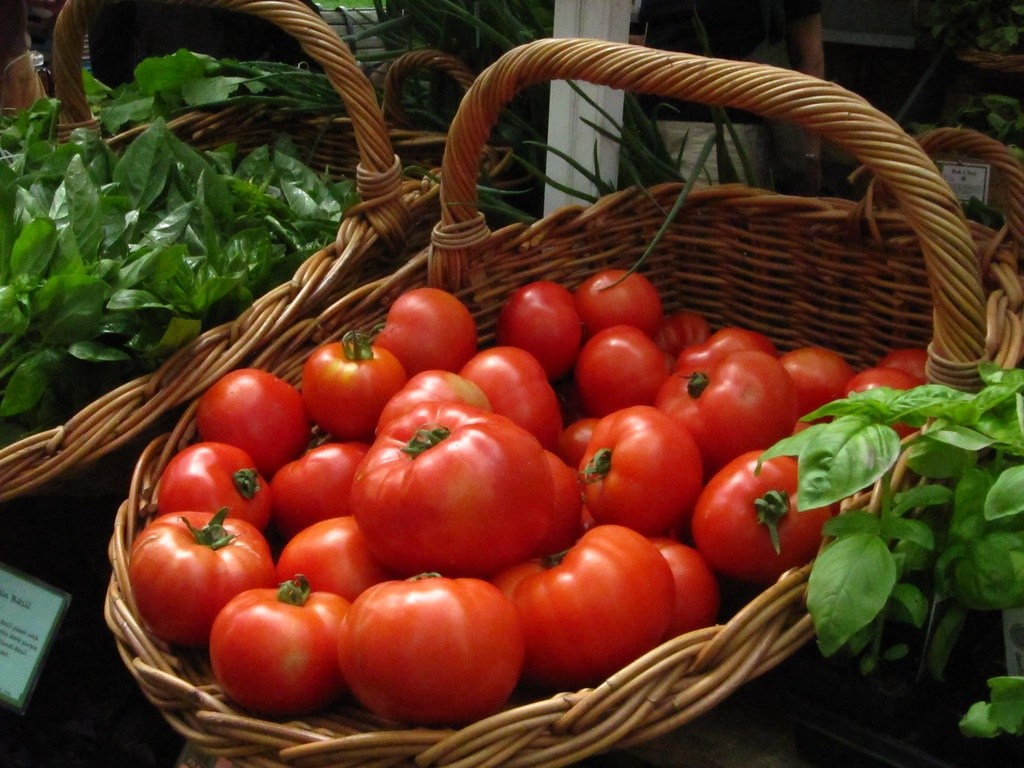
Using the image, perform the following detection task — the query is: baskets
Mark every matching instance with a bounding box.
[103,38,1024,768]
[1,0,511,522]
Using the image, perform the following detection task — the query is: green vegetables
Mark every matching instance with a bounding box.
[749,362,1024,743]
[0,48,376,455]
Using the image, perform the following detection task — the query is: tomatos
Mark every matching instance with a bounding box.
[128,270,927,725]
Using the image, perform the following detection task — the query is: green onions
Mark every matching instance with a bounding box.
[222,0,788,292]
